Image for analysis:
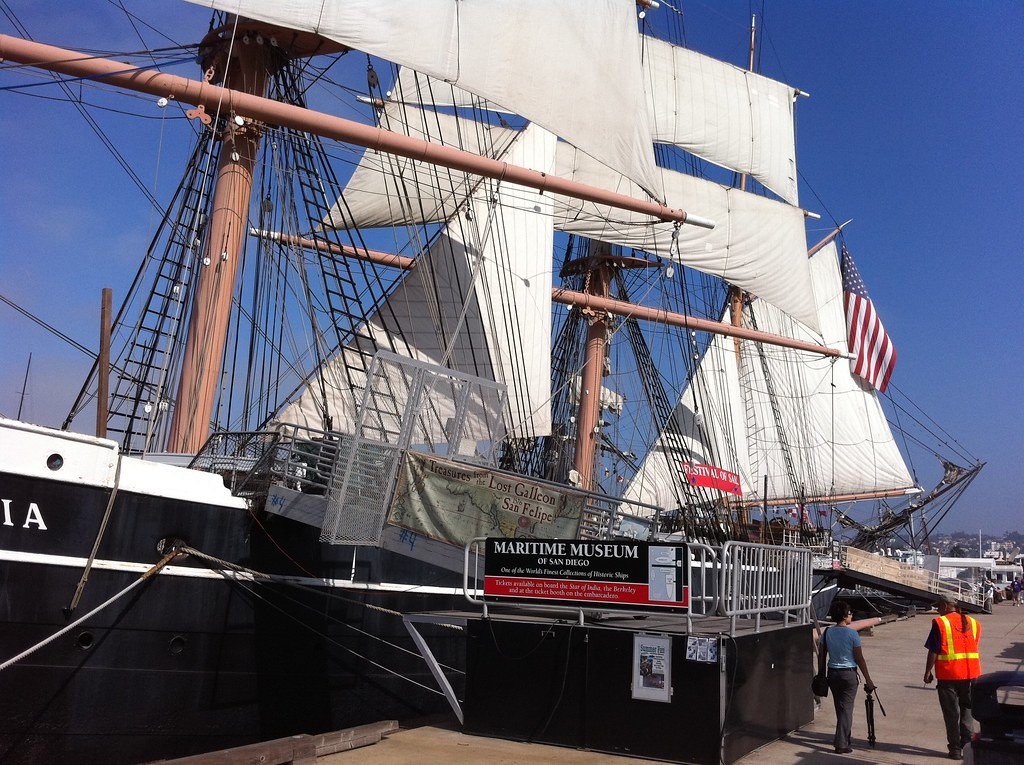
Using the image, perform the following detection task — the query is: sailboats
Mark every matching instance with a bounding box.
[0,1,1023,765]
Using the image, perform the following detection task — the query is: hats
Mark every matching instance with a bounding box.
[931,594,955,608]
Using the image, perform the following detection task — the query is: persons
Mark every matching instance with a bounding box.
[980,577,997,614]
[818,601,875,754]
[923,591,982,761]
[1011,577,1024,607]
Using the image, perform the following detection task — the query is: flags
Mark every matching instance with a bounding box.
[818,511,827,517]
[788,508,797,518]
[841,238,898,395]
[772,506,780,514]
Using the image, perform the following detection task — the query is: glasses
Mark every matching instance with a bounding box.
[848,610,851,615]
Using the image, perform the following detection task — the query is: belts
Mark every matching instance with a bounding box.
[828,667,856,671]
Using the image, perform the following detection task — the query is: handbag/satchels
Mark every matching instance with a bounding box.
[812,675,828,697]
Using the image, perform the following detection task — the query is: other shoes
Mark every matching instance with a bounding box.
[835,748,852,754]
[948,749,962,759]
[1017,604,1019,607]
[1013,602,1016,606]
